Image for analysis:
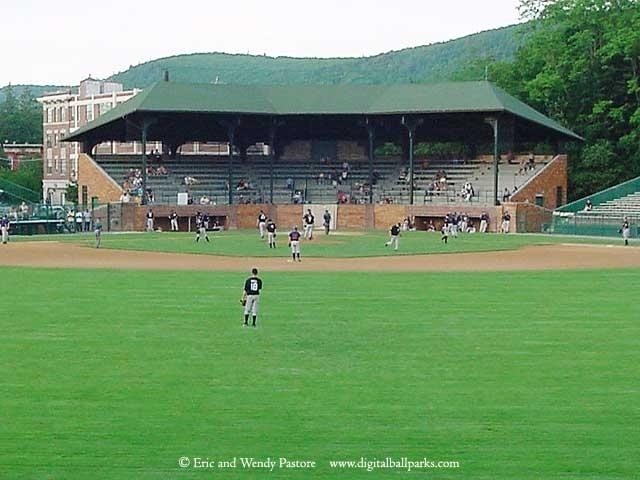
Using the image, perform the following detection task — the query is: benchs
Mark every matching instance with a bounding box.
[566,191,640,225]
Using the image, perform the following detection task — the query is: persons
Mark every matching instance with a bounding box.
[617,215,630,245]
[288,225,302,263]
[384,222,403,252]
[265,219,277,250]
[238,266,263,329]
[584,198,591,212]
[92,216,102,249]
[0,148,539,243]
